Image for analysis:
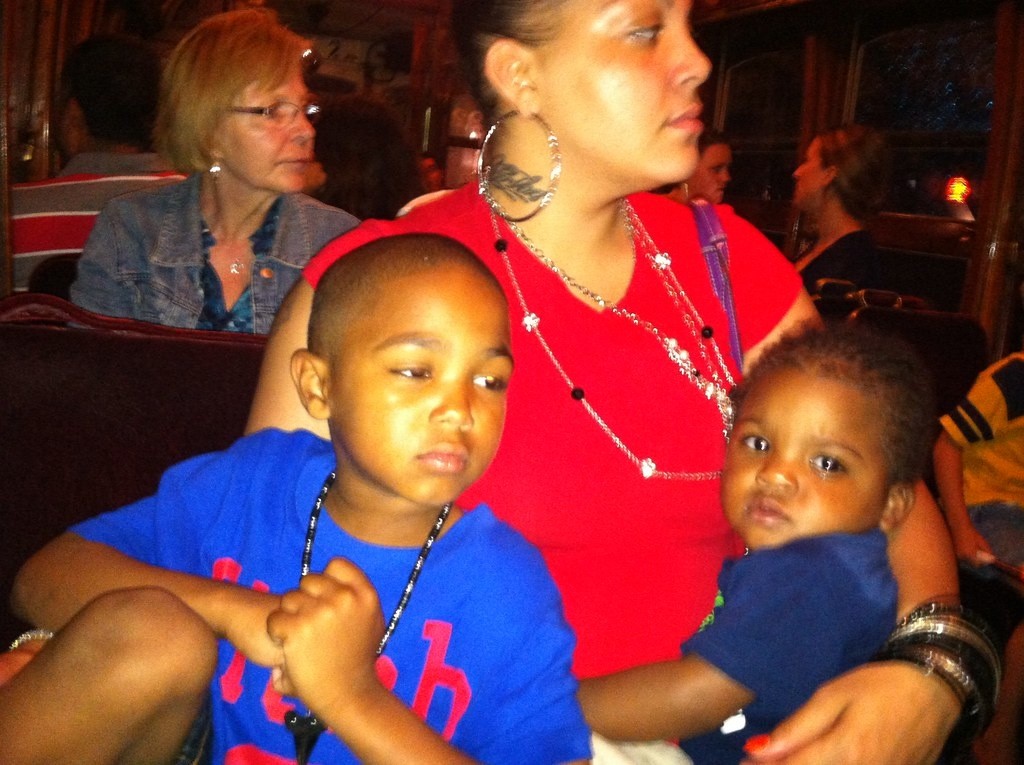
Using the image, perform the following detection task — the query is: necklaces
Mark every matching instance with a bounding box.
[228,256,244,276]
[284,467,452,765]
[483,195,739,480]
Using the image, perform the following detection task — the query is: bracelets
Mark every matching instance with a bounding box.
[886,599,999,741]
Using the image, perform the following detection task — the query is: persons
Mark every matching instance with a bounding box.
[932,352,1023,765]
[67,8,362,335]
[244,0,962,765]
[419,152,446,192]
[792,122,887,316]
[581,319,939,765]
[0,234,591,765]
[670,128,731,203]
[311,38,428,222]
[10,32,188,295]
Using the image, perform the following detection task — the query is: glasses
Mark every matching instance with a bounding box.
[221,97,322,125]
